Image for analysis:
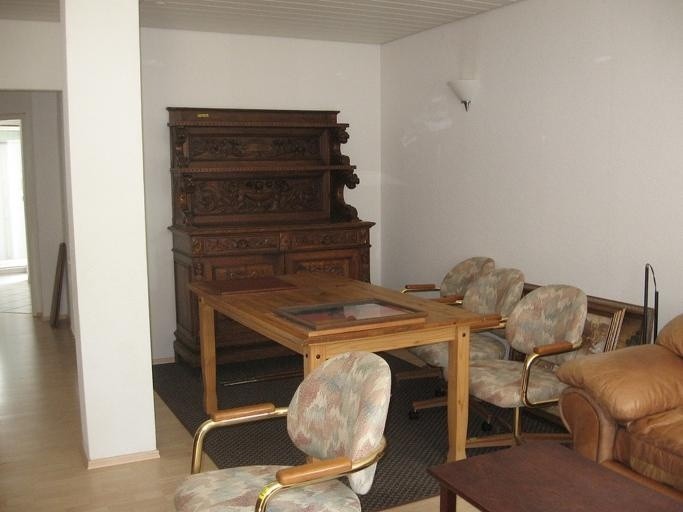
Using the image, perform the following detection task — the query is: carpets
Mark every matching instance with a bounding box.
[152,352,569,512]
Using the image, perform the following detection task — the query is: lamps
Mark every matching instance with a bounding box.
[447,79,478,112]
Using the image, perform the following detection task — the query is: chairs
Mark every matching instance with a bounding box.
[460,285,589,447]
[555,314,683,504]
[174,353,392,512]
[391,256,495,384]
[412,267,525,431]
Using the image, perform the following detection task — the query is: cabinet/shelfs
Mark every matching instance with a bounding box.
[166,106,376,380]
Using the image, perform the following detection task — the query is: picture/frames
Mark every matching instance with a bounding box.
[521,283,628,428]
[612,303,655,352]
[277,300,429,337]
[510,286,626,432]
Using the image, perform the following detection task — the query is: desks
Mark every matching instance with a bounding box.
[186,270,500,463]
